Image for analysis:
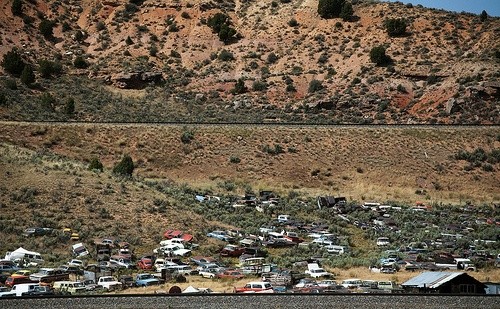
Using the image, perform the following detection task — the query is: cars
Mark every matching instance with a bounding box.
[0,189,500,295]
